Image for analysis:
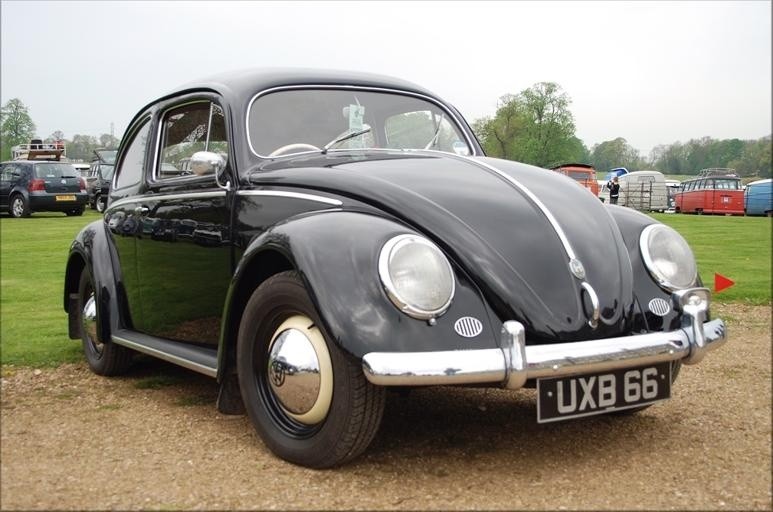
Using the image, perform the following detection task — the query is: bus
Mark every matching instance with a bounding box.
[543,160,772,218]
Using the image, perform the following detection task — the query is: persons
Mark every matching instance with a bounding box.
[606,176,619,205]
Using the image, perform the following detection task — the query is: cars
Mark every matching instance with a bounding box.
[61,64,733,473]
[274,356,297,376]
[105,211,226,248]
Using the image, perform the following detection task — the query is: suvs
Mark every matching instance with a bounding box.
[0,138,199,219]
